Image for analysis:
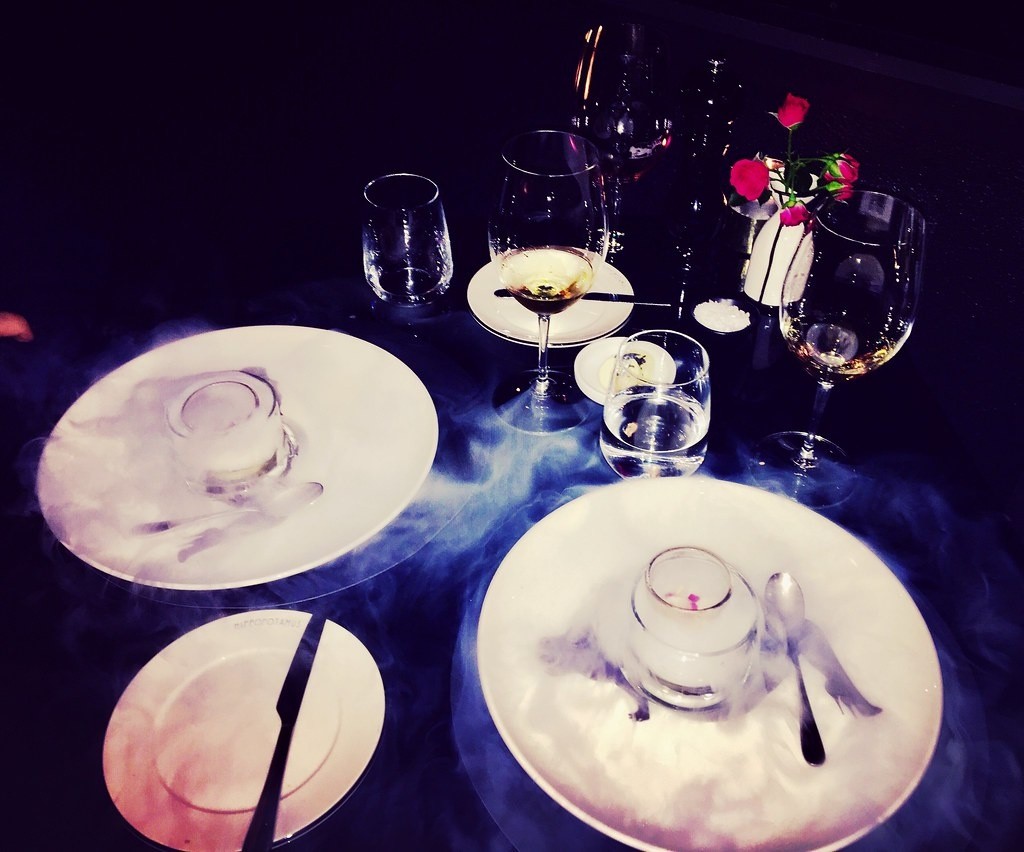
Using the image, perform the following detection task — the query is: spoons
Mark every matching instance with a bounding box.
[765,571,826,767]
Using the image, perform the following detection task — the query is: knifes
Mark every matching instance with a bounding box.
[495,289,673,306]
[241,606,329,852]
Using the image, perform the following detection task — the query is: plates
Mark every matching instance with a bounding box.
[467,244,637,350]
[35,322,440,592]
[573,336,677,407]
[100,607,386,852]
[474,474,945,852]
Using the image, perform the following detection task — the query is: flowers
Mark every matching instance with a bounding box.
[729,93,860,226]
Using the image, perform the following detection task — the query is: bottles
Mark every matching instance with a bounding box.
[683,56,737,171]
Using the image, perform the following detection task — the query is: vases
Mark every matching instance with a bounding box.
[744,165,820,306]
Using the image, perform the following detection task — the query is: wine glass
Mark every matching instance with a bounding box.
[485,128,614,436]
[693,106,825,334]
[748,189,928,511]
[361,173,454,348]
[573,23,676,270]
[598,328,713,484]
